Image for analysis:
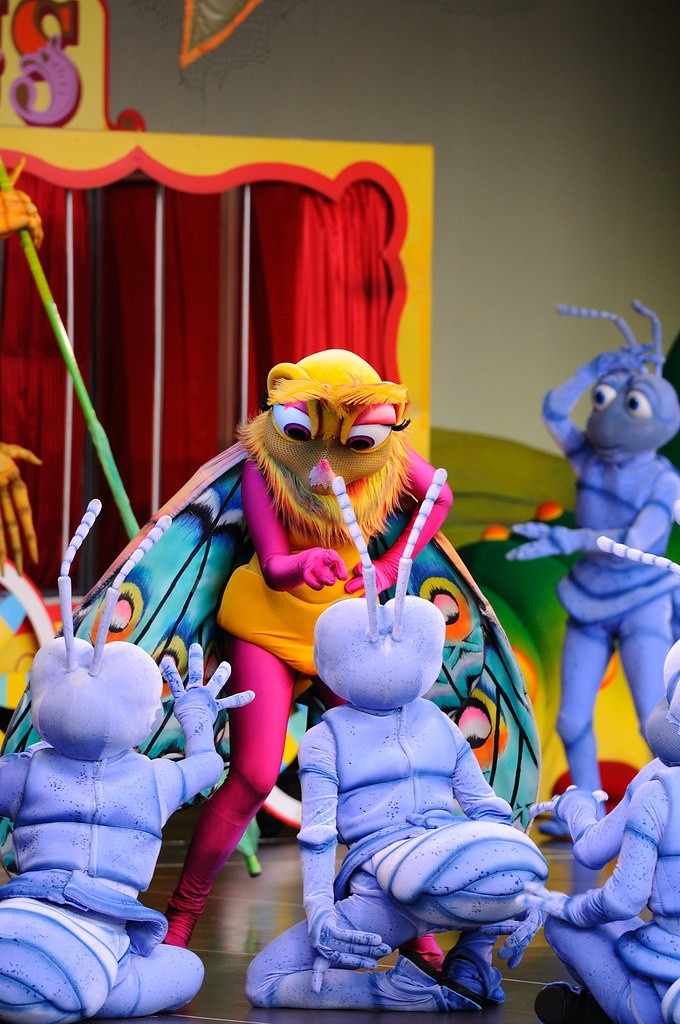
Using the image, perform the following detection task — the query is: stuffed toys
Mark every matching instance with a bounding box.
[245,467,546,1010]
[162,350,453,971]
[0,498,255,1024]
[518,638,680,1024]
[506,298,680,837]
